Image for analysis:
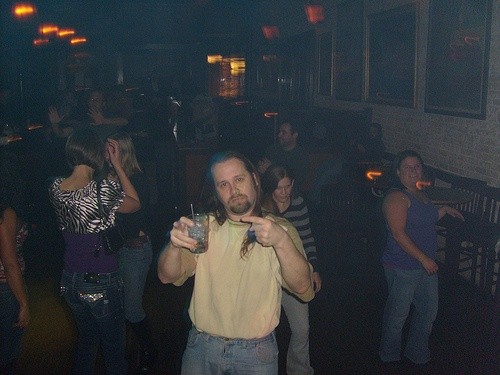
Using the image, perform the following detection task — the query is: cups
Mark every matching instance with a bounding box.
[186,214,209,254]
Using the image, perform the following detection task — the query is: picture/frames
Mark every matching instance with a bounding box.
[423,0,492,119]
[366,2,422,109]
[336,1,366,103]
[317,32,334,95]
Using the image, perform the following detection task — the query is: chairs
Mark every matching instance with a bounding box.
[437,177,500,298]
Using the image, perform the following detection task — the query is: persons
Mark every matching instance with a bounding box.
[0,88,464,375]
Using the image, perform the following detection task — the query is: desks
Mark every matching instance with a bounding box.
[426,187,471,206]
